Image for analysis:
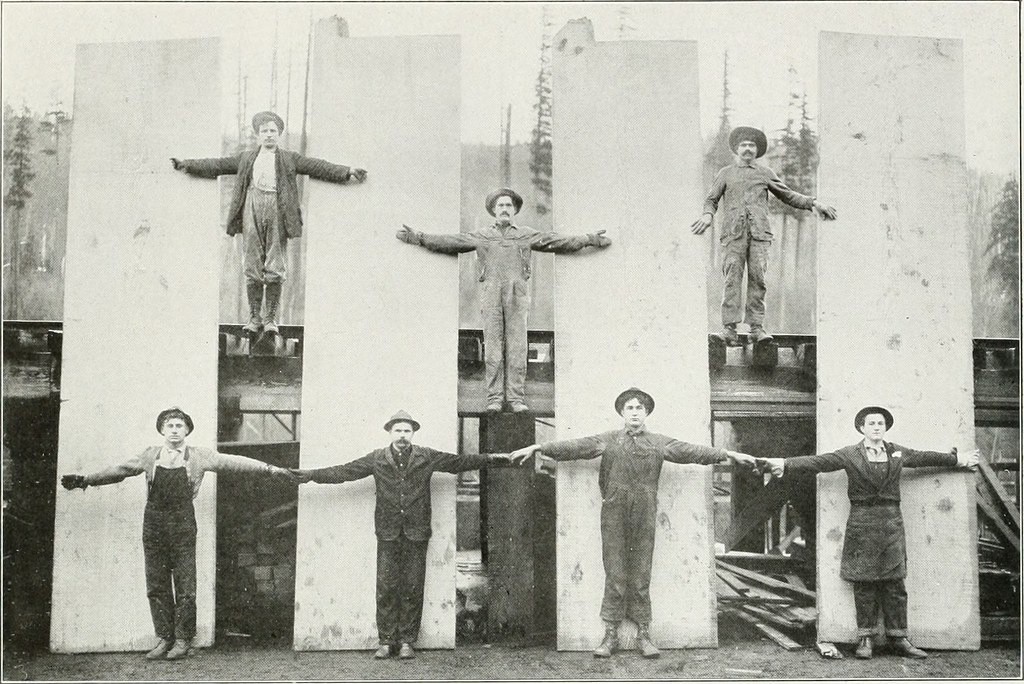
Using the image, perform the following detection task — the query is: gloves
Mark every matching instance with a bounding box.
[955,450,980,473]
[750,457,785,479]
[489,453,513,466]
[395,223,423,247]
[61,474,88,490]
[269,467,294,484]
[288,467,314,483]
[586,228,611,248]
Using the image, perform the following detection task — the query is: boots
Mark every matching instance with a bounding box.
[263,280,280,334]
[241,280,262,332]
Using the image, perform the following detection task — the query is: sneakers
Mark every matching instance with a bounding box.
[708,325,739,346]
[485,399,507,413]
[854,636,875,660]
[146,637,172,660]
[375,643,391,659]
[168,638,192,660]
[503,400,529,413]
[635,632,660,659]
[747,325,776,343]
[398,642,415,659]
[892,636,928,658]
[594,629,620,658]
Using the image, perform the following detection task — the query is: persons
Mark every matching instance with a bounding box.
[61,408,296,660]
[508,386,758,658]
[397,188,612,412]
[291,410,513,659]
[170,111,367,335]
[758,406,980,659]
[691,126,836,345]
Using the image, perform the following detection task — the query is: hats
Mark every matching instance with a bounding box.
[613,386,656,418]
[251,110,285,138]
[484,187,523,219]
[728,126,767,159]
[384,410,420,432]
[852,406,893,436]
[155,404,194,433]
